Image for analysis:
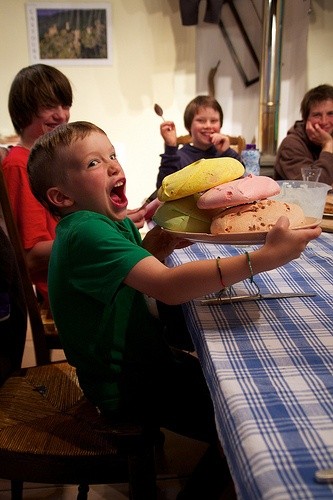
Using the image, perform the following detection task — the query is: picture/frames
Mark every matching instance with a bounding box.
[26,2,112,68]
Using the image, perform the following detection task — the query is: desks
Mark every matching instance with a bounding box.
[154,220,333,500]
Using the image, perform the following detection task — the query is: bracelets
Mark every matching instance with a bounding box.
[214,256,238,310]
[244,251,263,298]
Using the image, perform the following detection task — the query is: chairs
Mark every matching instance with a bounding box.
[0,174,166,500]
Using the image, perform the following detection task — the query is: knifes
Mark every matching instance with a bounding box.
[198,293,316,305]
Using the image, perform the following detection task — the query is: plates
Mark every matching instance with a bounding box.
[322,213,333,216]
[159,214,322,244]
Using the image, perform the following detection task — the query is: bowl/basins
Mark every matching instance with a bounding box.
[267,179,332,220]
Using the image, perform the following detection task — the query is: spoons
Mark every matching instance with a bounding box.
[154,103,165,121]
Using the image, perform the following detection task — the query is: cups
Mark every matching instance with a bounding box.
[301,166,322,182]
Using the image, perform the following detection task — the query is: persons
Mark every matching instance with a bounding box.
[156,95,246,189]
[3,64,160,318]
[274,85,333,186]
[27,121,319,444]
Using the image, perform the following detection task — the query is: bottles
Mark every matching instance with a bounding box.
[241,143,261,176]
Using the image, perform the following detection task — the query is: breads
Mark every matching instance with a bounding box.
[151,157,306,235]
[323,202,333,214]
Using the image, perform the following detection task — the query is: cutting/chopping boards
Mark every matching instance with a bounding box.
[317,218,333,233]
